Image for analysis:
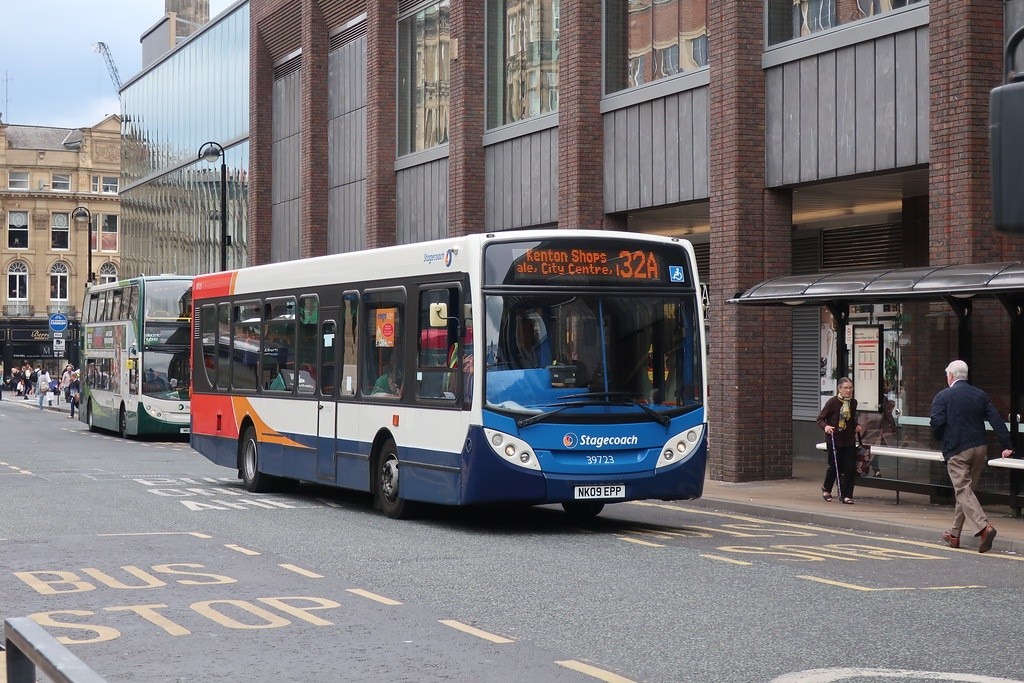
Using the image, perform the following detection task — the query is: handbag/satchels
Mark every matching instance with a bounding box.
[854,424,871,478]
[17,381,21,390]
[74,390,80,403]
[40,381,49,392]
[20,385,23,392]
[45,391,54,401]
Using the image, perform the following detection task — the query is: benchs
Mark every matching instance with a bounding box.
[816,442,1024,518]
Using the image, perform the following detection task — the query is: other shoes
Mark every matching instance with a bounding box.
[15,394,19,396]
[24,396,28,399]
[66,415,74,419]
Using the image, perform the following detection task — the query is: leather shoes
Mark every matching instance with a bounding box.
[978,525,997,553]
[942,532,959,548]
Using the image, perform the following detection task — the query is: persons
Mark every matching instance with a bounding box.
[855,411,888,477]
[817,377,861,504]
[0,364,80,419]
[371,343,403,398]
[930,359,1012,553]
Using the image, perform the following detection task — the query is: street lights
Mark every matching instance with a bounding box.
[70,206,93,288]
[198,141,227,270]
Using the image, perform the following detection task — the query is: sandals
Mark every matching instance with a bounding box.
[841,497,854,504]
[821,484,832,502]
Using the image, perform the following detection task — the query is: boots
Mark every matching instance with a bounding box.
[49,401,52,406]
[40,403,43,410]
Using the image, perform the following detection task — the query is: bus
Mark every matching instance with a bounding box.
[186,227,711,519]
[76,273,200,442]
